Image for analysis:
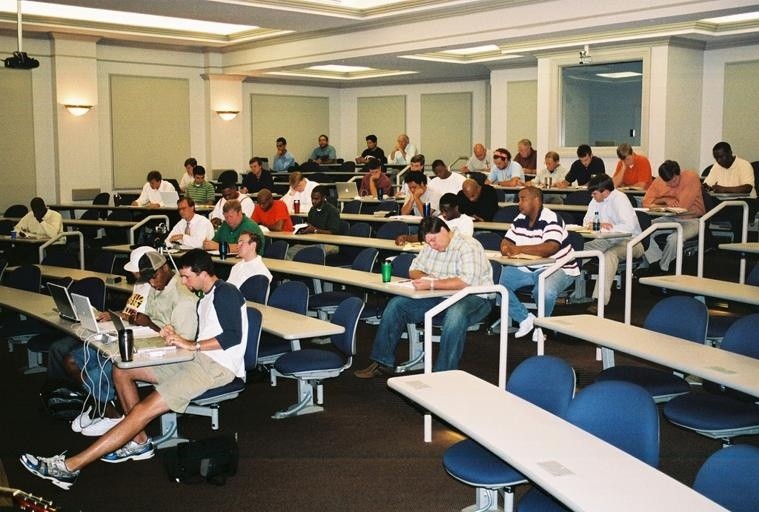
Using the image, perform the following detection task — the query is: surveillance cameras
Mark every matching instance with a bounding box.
[579,44,593,64]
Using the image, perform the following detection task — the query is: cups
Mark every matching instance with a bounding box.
[423,201,430,217]
[118,328,135,363]
[10,231,17,238]
[294,199,301,214]
[219,240,227,260]
[544,176,552,188]
[379,259,393,282]
[114,194,120,207]
[377,187,383,201]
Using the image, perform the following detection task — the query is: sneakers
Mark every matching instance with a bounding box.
[354,363,392,378]
[82,415,125,436]
[100,440,154,463]
[532,328,546,342]
[20,453,80,490]
[515,313,538,339]
[72,399,116,432]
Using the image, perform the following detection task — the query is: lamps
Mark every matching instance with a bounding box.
[217,110,237,122]
[63,104,93,118]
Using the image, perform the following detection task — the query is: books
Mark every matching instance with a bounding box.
[389,214,421,220]
[565,224,615,235]
[291,223,310,235]
[391,277,439,291]
[648,206,688,214]
[97,320,161,341]
[504,252,542,261]
[129,336,176,353]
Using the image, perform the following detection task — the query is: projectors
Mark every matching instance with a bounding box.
[4,51,39,69]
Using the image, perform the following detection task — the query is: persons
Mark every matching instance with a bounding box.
[387,134,417,165]
[513,139,537,174]
[131,170,179,208]
[278,172,326,212]
[457,179,499,222]
[359,160,394,196]
[250,188,293,232]
[565,144,605,187]
[522,152,568,188]
[428,159,468,196]
[19,248,248,491]
[226,230,272,290]
[461,144,494,173]
[272,137,295,172]
[46,245,155,412]
[356,135,385,163]
[355,217,496,378]
[309,135,337,165]
[612,143,653,190]
[498,186,581,342]
[581,174,644,313]
[240,156,275,194]
[180,158,197,193]
[13,196,68,245]
[485,148,525,203]
[396,153,431,197]
[166,198,215,247]
[208,180,255,230]
[299,185,340,235]
[702,142,759,255]
[395,193,475,246]
[70,251,200,436]
[180,165,215,205]
[203,199,265,257]
[642,159,706,276]
[400,170,442,218]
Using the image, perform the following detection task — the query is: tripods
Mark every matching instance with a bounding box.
[155,249,178,271]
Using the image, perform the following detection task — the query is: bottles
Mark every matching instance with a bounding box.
[594,212,601,232]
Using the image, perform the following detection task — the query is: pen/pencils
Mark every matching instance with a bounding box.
[399,279,413,284]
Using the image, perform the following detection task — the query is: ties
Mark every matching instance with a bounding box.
[184,223,190,235]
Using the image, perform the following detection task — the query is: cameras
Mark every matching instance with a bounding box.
[155,224,170,240]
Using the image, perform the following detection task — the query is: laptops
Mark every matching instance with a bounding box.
[160,192,181,208]
[109,310,158,336]
[260,157,270,171]
[72,293,128,331]
[47,283,99,321]
[336,182,359,198]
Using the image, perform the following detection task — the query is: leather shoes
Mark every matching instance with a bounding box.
[588,299,601,314]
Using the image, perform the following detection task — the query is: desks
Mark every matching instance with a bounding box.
[487,180,744,199]
[269,170,375,177]
[387,368,731,512]
[288,211,487,229]
[214,192,284,197]
[639,274,758,308]
[7,263,346,341]
[285,211,633,241]
[1,285,195,370]
[104,244,463,300]
[719,242,759,253]
[1,216,142,227]
[1,234,51,243]
[532,315,758,399]
[316,162,415,167]
[356,194,690,218]
[210,179,341,188]
[213,230,556,253]
[48,202,212,211]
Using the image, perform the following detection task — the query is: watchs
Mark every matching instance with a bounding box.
[313,228,319,233]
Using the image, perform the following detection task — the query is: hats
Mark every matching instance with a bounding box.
[124,246,157,272]
[139,252,167,272]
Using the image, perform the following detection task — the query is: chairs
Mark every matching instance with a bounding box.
[563,229,595,308]
[341,200,362,214]
[750,161,759,204]
[653,193,713,258]
[516,380,660,512]
[0,265,52,375]
[662,315,759,441]
[69,276,107,312]
[391,253,417,375]
[350,223,372,238]
[417,262,503,372]
[623,191,647,207]
[340,219,349,236]
[261,240,289,260]
[442,356,576,512]
[376,221,409,241]
[564,190,594,205]
[240,275,269,306]
[475,231,503,252]
[291,245,325,320]
[270,297,365,421]
[701,164,714,181]
[556,210,587,232]
[150,306,263,450]
[554,208,585,229]
[309,247,379,346]
[691,444,759,512]
[3,204,28,220]
[591,295,708,404]
[258,281,310,388]
[613,210,650,295]
[375,200,400,216]
[493,205,522,223]
[743,260,759,286]
[498,204,524,225]
